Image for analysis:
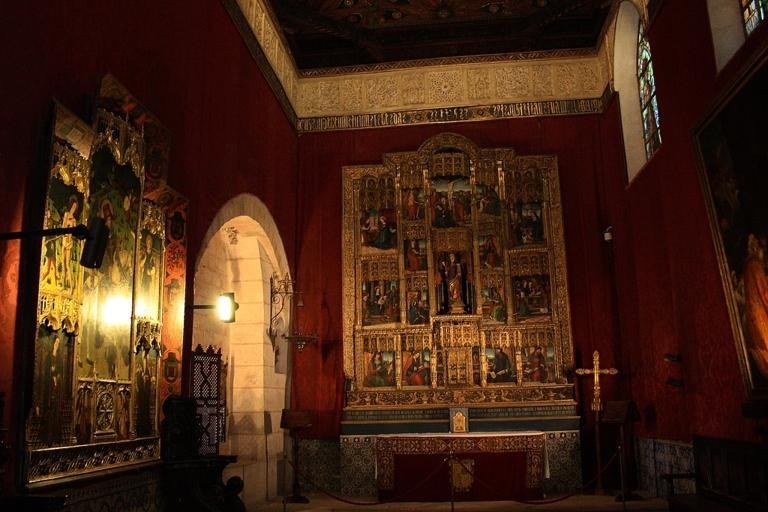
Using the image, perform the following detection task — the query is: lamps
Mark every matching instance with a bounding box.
[1,219,111,270]
[581,417,621,494]
[193,293,240,323]
[280,409,314,504]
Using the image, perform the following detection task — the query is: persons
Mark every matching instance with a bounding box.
[741,233,768,378]
[361,191,547,385]
[34,194,160,445]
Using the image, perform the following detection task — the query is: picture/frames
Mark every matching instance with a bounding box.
[685,36,767,429]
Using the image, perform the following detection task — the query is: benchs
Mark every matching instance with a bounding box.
[661,433,767,511]
